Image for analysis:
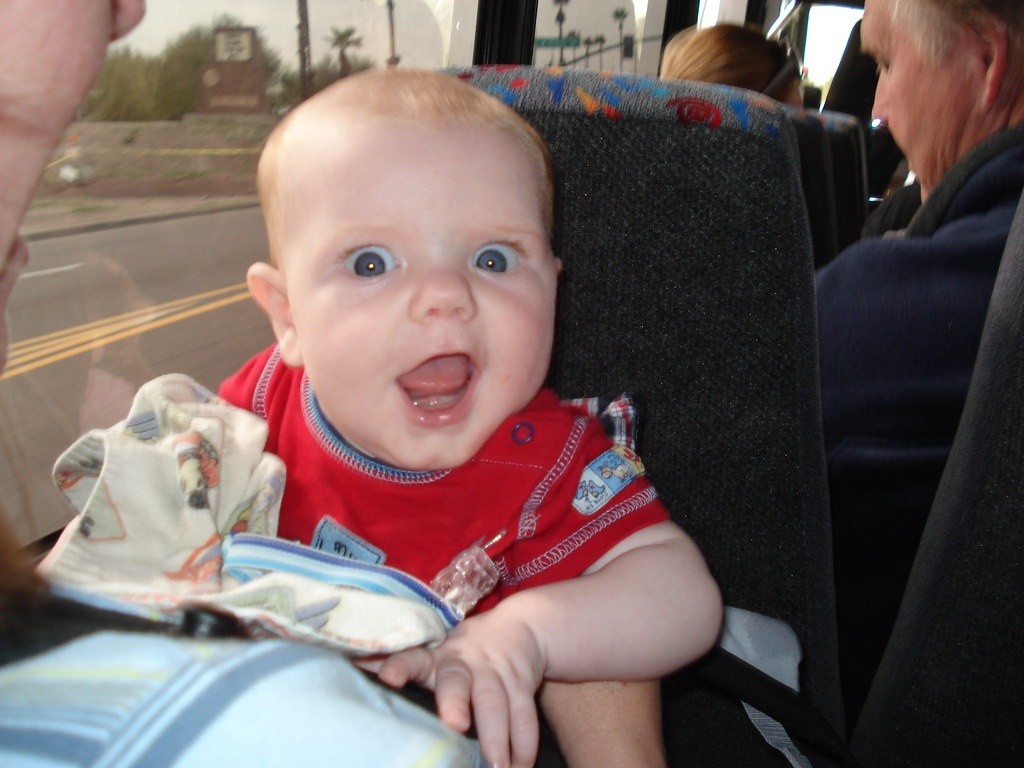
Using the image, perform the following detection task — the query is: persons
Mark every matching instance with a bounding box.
[219,65,726,768]
[0,0,1024,768]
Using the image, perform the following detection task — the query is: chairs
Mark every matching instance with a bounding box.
[430,63,1024,768]
